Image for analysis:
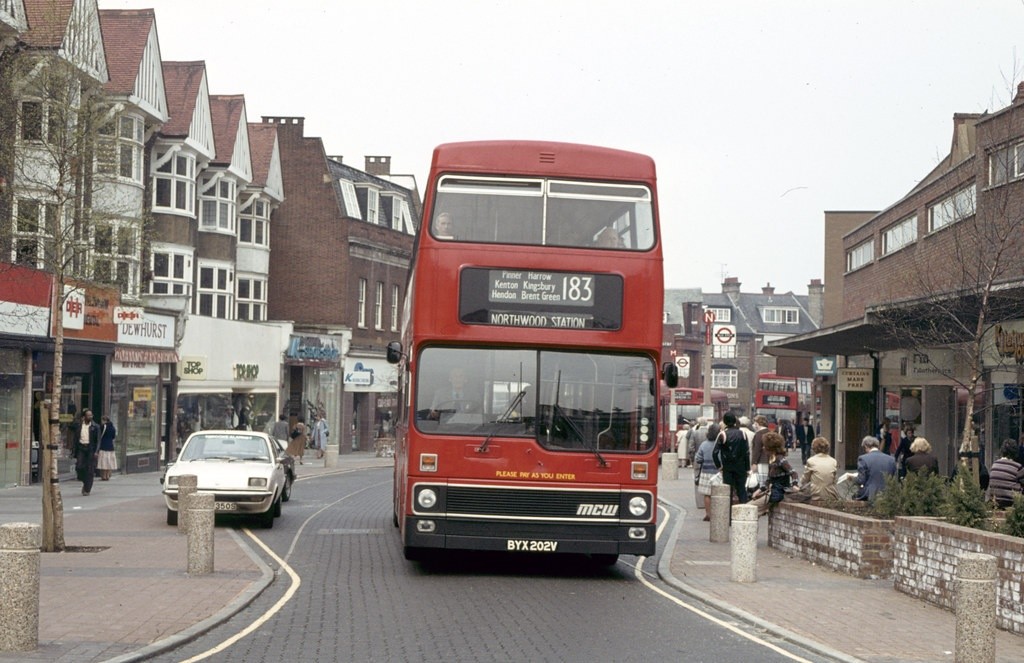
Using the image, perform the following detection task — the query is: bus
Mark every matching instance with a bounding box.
[750,372,821,436]
[659,387,730,453]
[386,141,679,577]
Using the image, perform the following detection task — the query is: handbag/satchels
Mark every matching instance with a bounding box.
[708,472,723,485]
[745,472,759,488]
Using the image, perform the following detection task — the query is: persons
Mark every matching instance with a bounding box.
[286,416,308,465]
[676,417,727,468]
[74,409,101,497]
[796,418,815,469]
[694,423,722,521]
[425,365,477,420]
[713,411,750,504]
[750,416,769,497]
[847,435,896,501]
[747,432,800,517]
[876,423,939,481]
[235,407,249,431]
[799,437,837,501]
[596,227,618,248]
[435,213,455,239]
[768,418,796,451]
[738,415,755,481]
[95,416,118,481]
[311,412,329,450]
[273,415,289,451]
[989,437,1024,504]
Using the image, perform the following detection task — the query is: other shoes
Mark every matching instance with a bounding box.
[82,487,90,496]
[703,515,710,522]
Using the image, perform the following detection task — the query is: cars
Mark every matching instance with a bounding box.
[163,430,298,530]
